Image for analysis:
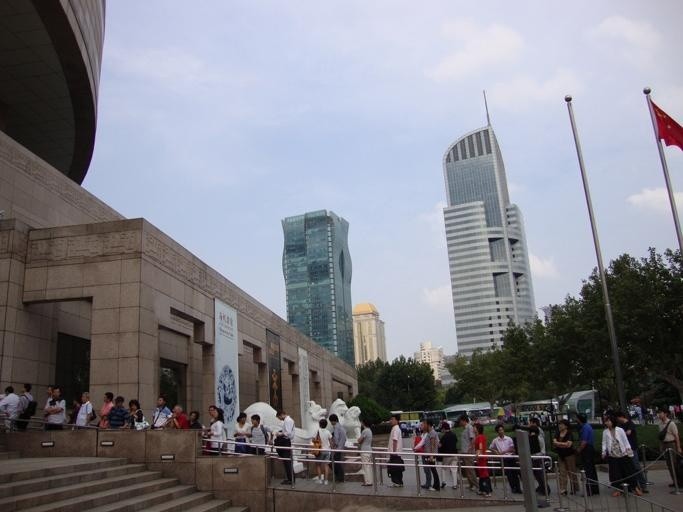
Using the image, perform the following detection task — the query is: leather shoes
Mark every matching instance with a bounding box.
[612,491,621,497]
[635,490,644,496]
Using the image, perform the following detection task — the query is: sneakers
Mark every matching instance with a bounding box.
[441,483,446,489]
[316,477,329,485]
[466,486,492,498]
[452,486,457,489]
[421,484,440,491]
[387,482,403,489]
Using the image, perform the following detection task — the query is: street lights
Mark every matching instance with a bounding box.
[639,368,651,425]
[595,383,606,427]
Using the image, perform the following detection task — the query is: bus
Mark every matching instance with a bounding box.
[424,410,443,432]
[508,401,569,428]
[442,407,505,429]
[371,410,424,432]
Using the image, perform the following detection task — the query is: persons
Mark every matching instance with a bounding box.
[275,412,403,488]
[401,403,683,496]
[1,384,144,435]
[150,396,274,456]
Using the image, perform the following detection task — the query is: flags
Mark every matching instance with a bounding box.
[651,99,683,150]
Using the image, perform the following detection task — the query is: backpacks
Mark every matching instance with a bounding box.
[250,425,269,444]
[18,395,38,417]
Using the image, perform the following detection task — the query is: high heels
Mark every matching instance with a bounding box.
[559,490,568,496]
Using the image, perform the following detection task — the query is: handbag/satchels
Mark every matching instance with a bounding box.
[656,419,672,442]
[386,455,405,478]
[85,404,97,423]
[133,409,150,430]
[311,430,322,456]
